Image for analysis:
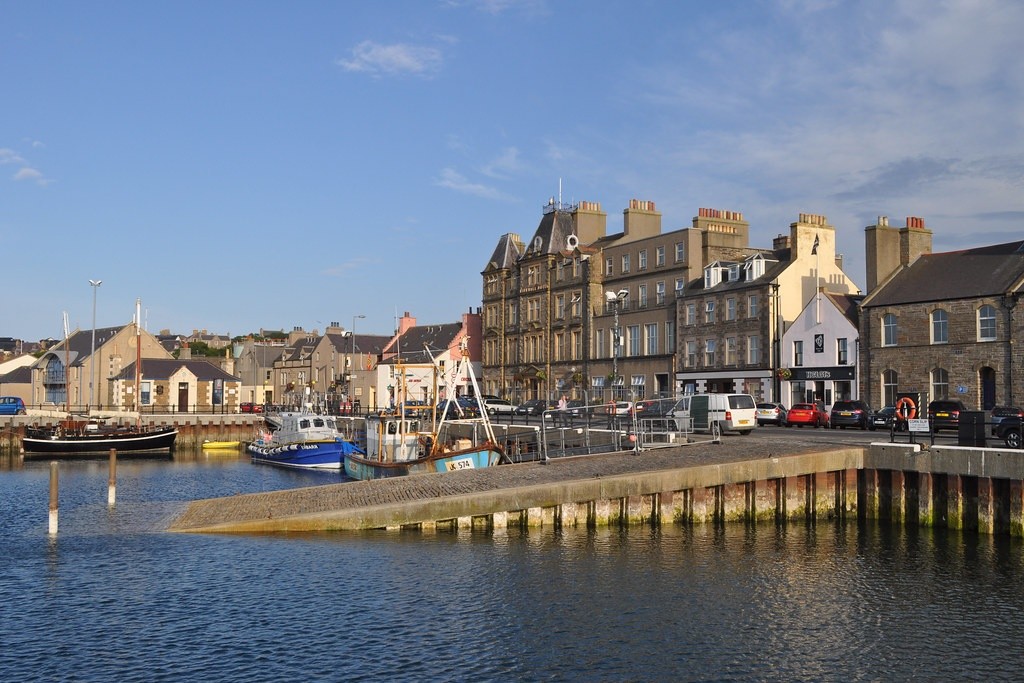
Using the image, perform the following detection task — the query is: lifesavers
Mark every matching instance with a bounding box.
[895,397,916,421]
[605,400,616,416]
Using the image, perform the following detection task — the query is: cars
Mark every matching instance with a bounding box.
[606,401,634,416]
[482,395,519,415]
[514,400,557,417]
[755,402,788,427]
[991,404,1024,435]
[997,417,1024,449]
[396,400,430,417]
[831,399,874,430]
[929,399,966,433]
[240,402,263,414]
[870,406,906,432]
[437,399,481,420]
[0,396,26,415]
[628,401,676,426]
[786,403,830,429]
[567,400,594,418]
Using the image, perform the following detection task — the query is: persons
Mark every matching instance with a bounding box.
[559,395,568,426]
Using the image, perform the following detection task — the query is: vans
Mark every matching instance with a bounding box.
[664,394,758,436]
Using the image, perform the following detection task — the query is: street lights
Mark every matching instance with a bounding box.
[353,315,366,417]
[605,290,629,430]
[89,280,102,411]
[768,293,783,404]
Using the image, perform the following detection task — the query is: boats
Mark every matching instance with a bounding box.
[248,392,356,475]
[202,439,240,449]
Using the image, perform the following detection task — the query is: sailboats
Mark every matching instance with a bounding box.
[22,298,180,456]
[344,304,507,481]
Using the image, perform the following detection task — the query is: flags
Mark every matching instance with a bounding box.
[811,234,819,254]
